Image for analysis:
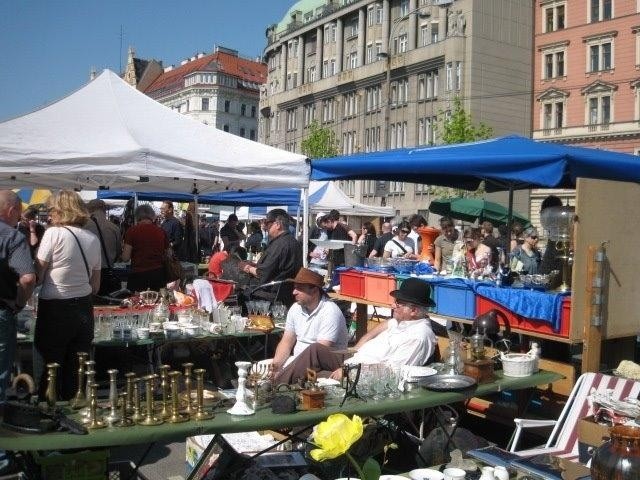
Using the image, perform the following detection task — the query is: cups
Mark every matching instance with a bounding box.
[351,361,400,402]
[323,384,347,400]
[443,468,467,480]
[136,299,243,341]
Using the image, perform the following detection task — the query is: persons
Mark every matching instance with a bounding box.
[307,209,542,353]
[0,187,303,397]
[258,277,436,397]
[267,267,349,381]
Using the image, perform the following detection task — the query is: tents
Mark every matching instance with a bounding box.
[266,181,396,241]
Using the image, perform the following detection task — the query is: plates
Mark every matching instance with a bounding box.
[402,365,438,378]
[180,389,218,403]
[419,374,477,391]
[409,468,444,480]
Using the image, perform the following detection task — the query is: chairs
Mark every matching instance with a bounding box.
[511,370,640,470]
[248,277,295,306]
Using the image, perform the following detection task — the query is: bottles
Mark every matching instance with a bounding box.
[477,466,510,480]
[93,313,131,342]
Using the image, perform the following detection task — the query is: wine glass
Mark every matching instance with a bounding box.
[245,300,286,331]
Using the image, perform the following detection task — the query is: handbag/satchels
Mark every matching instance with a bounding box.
[157,254,184,280]
[100,267,121,297]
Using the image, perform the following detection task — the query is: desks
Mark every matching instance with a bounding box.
[1,357,566,480]
[113,259,209,271]
[384,457,590,480]
[14,313,287,391]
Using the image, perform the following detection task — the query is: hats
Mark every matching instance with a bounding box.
[390,278,437,308]
[289,268,331,299]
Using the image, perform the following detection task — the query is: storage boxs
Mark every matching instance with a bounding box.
[339,268,571,338]
[23,447,111,480]
[184,428,293,480]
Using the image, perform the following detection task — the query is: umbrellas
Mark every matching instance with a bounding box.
[309,134,640,266]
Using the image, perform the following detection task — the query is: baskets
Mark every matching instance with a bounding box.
[502,353,535,378]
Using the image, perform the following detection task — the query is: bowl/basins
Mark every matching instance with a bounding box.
[18,306,35,321]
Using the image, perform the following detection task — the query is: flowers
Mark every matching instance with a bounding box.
[308,412,381,480]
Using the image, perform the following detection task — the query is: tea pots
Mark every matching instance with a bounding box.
[463,327,488,362]
[440,328,466,375]
